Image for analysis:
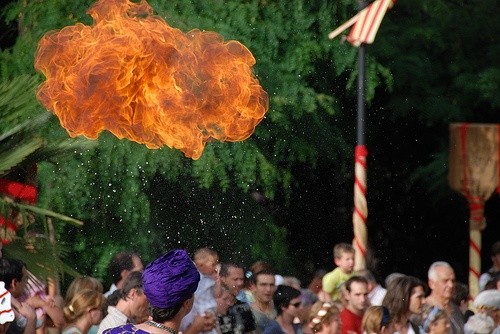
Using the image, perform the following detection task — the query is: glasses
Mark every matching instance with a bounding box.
[288,301,302,308]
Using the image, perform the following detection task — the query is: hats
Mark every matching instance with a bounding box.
[142,250,200,307]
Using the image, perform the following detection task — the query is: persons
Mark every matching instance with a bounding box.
[236,244,500,333]
[0,248,257,334]
